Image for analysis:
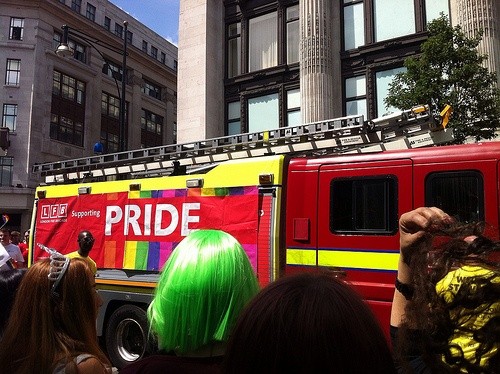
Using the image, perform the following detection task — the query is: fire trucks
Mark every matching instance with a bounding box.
[25,104,500,371]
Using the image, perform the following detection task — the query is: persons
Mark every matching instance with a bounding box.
[0,227,30,270]
[62,230,97,282]
[0,256,114,374]
[118,229,259,374]
[429,234,500,374]
[0,268,30,336]
[387,206,500,374]
[220,271,394,374]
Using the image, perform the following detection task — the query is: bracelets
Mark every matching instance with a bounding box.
[394,274,431,301]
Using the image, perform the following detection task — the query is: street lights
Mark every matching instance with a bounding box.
[54,21,130,158]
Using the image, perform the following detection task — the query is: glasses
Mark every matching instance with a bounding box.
[77,237,95,243]
